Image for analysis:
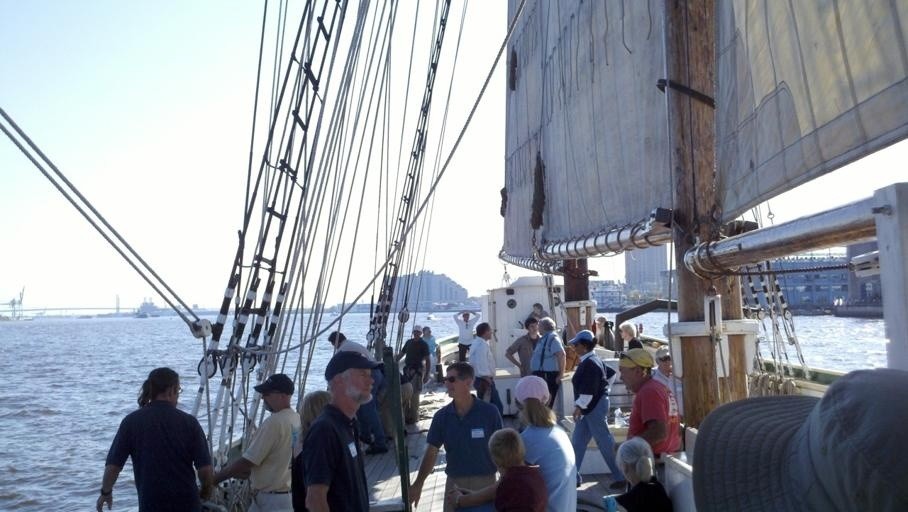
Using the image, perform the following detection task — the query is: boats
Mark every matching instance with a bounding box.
[135,312,160,319]
[595,306,625,313]
[427,313,441,321]
[830,302,883,318]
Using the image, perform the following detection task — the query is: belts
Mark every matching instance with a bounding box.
[459,344,472,350]
[270,491,288,494]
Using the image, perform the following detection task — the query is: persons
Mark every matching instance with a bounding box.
[407,361,505,512]
[214,374,307,511]
[568,329,625,490]
[619,348,682,455]
[97,367,216,512]
[397,301,685,415]
[303,351,385,510]
[328,329,387,456]
[292,388,339,510]
[445,426,546,510]
[577,437,671,512]
[514,377,577,512]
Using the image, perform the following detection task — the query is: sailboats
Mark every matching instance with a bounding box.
[189,0,906,512]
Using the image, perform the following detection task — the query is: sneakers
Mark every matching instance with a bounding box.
[609,480,631,489]
[365,446,387,454]
[358,432,371,444]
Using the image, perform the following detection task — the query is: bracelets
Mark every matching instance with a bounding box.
[456,493,461,506]
[100,489,113,495]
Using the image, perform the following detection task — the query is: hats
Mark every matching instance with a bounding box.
[412,326,422,334]
[655,344,671,359]
[618,348,655,368]
[693,368,907,511]
[514,374,550,406]
[569,330,595,344]
[254,374,295,394]
[325,351,385,380]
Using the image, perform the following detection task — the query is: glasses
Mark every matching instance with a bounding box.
[618,351,640,367]
[178,387,183,394]
[443,376,455,383]
